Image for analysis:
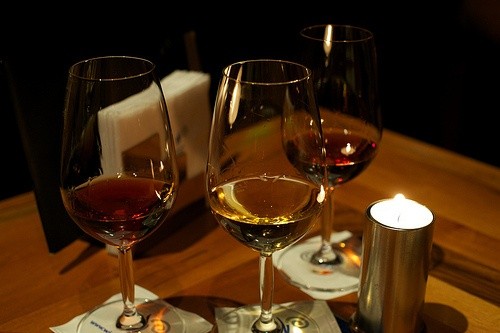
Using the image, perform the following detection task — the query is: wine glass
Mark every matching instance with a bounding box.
[60,56,184,333]
[277,26,384,292]
[206,60,326,333]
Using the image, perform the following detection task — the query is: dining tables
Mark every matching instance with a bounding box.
[0,103,500,333]
